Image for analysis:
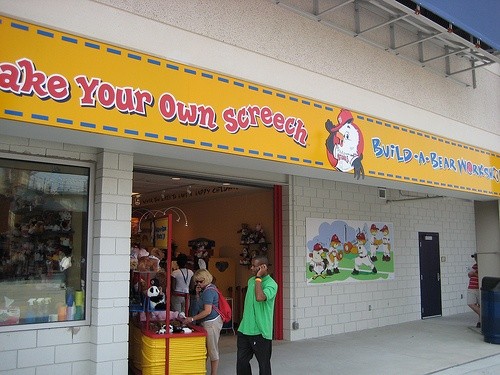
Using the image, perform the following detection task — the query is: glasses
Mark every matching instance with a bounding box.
[196,280,204,283]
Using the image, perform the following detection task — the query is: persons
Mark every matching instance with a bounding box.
[466,252,481,328]
[236,255,279,375]
[171,250,230,375]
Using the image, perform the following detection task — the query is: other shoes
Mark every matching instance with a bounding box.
[476,323,480,327]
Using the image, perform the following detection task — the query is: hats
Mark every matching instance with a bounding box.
[471,253,477,258]
[198,259,206,269]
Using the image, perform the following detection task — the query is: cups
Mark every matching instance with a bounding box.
[75,292,83,306]
[74,305,85,319]
[67,307,75,320]
[57,306,67,321]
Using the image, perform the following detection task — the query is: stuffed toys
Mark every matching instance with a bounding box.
[1,212,72,277]
[236,223,268,264]
[131,241,215,334]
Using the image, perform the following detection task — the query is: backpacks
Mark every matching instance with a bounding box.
[203,287,232,323]
